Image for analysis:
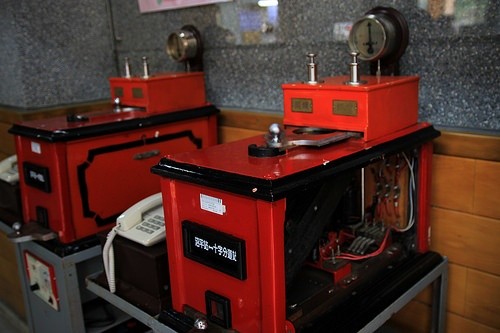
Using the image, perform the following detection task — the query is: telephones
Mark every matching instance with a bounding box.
[0,154,20,183]
[116,191,167,247]
